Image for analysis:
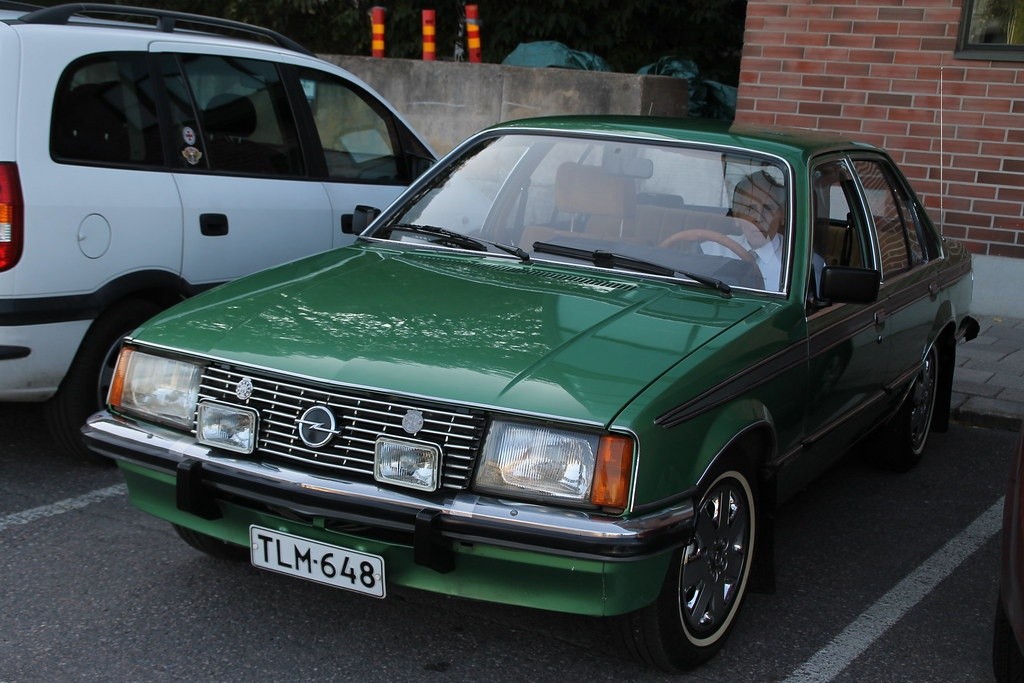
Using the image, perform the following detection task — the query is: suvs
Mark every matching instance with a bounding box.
[0,0,548,469]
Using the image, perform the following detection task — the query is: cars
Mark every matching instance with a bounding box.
[991,411,1024,683]
[77,117,979,669]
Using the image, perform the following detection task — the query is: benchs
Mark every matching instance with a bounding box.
[587,203,861,266]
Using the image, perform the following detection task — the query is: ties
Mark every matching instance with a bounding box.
[749,250,757,258]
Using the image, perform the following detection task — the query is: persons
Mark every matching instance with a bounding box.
[674,170,823,304]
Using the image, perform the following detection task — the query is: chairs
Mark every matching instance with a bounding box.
[73,108,129,161]
[520,161,630,253]
[204,94,288,176]
